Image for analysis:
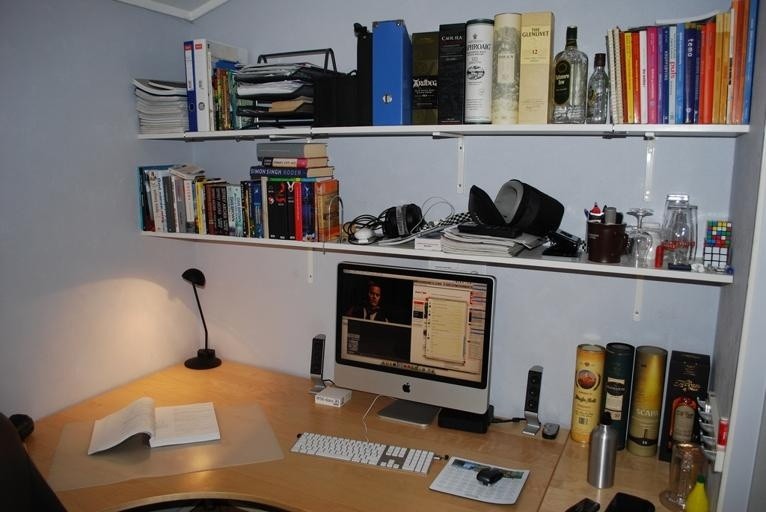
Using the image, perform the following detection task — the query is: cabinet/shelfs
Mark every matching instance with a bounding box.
[133,119,750,283]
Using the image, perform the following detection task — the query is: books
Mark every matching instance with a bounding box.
[605,0,761,125]
[138,142,340,241]
[131,38,340,133]
[85,396,223,458]
[377,220,549,258]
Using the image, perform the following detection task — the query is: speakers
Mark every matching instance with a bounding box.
[522,365,544,435]
[308,334,326,394]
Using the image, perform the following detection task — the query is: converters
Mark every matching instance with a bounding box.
[436,402,495,435]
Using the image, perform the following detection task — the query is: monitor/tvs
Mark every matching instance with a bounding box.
[334,260,498,427]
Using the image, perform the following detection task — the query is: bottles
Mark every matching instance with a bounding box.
[569,344,605,445]
[627,345,666,457]
[491,12,520,126]
[685,474,711,510]
[602,342,634,453]
[462,16,493,123]
[718,415,729,445]
[669,384,700,451]
[555,24,588,126]
[589,53,609,124]
[586,411,617,490]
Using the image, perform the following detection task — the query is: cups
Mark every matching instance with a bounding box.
[624,194,700,268]
[584,221,626,264]
[665,443,705,508]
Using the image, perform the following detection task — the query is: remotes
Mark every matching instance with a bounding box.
[543,423,560,439]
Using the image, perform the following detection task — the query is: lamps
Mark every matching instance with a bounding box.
[181,268,223,370]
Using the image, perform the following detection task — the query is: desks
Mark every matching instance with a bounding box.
[537,432,676,511]
[21,358,570,511]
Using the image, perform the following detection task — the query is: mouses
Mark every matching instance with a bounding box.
[476,467,504,485]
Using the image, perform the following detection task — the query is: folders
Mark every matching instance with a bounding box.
[372,20,412,125]
[183,38,246,131]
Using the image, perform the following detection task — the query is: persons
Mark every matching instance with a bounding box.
[349,282,388,323]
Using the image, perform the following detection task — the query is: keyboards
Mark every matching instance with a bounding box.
[289,431,435,474]
